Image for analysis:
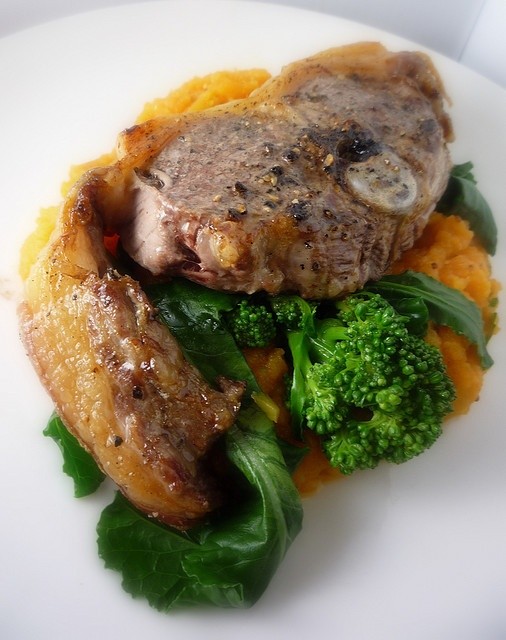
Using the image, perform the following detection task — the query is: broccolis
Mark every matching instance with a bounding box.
[231,294,456,474]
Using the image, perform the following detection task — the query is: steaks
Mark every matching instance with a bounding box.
[123,50,464,301]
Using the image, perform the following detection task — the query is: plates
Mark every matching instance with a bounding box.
[0,0,505,638]
